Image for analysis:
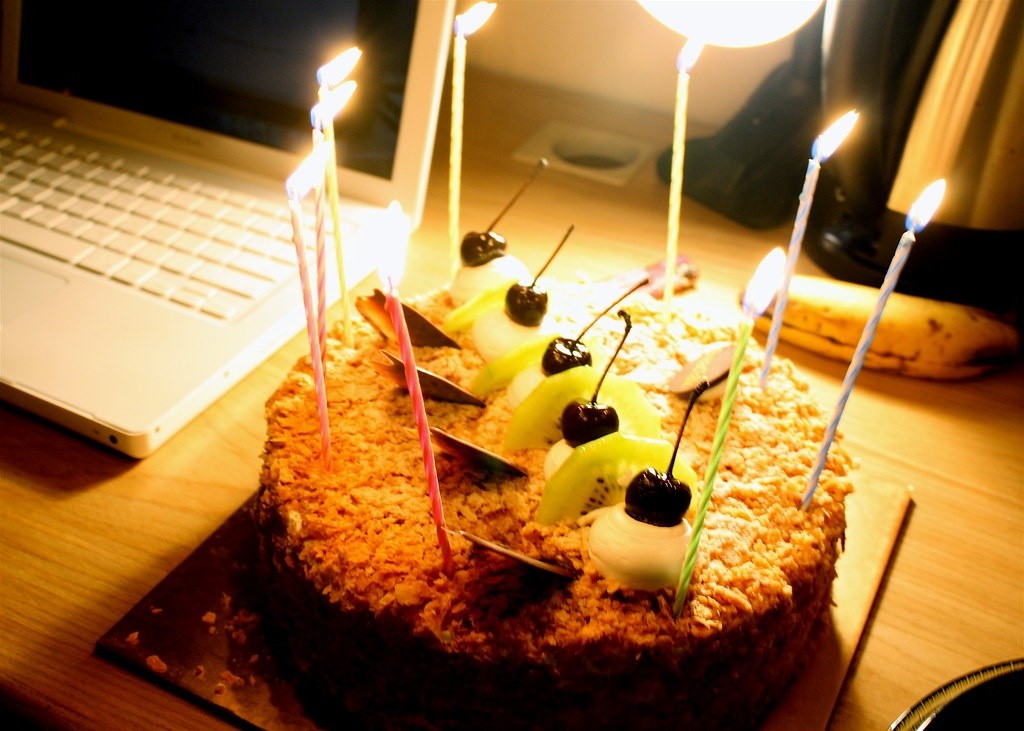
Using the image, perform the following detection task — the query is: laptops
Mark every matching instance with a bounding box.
[3,0,454,459]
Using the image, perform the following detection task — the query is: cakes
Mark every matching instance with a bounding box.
[251,155,859,731]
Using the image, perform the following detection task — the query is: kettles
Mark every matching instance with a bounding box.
[811,0,1024,326]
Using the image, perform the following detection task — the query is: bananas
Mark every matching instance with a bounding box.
[741,274,1016,384]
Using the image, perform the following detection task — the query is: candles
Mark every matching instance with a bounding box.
[671,247,787,617]
[660,38,707,304]
[448,0,497,277]
[316,44,364,347]
[284,142,334,471]
[798,179,947,509]
[753,107,862,388]
[309,79,358,391]
